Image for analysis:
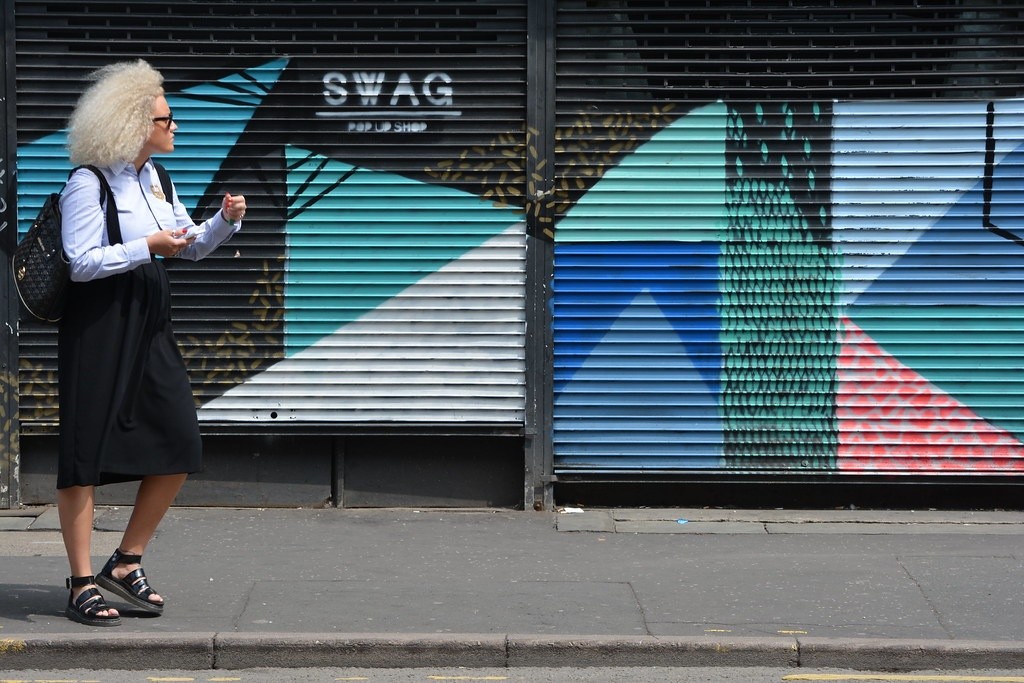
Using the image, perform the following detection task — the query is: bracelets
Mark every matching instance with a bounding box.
[225,211,247,225]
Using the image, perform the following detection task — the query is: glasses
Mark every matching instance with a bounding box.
[154,112,173,127]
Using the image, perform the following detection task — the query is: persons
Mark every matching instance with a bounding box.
[53,58,246,624]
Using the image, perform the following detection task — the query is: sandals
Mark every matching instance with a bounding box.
[94,548,164,614]
[65,576,122,627]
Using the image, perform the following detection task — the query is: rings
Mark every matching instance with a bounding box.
[171,230,176,237]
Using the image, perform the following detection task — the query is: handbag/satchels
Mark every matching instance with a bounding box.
[12,165,107,323]
